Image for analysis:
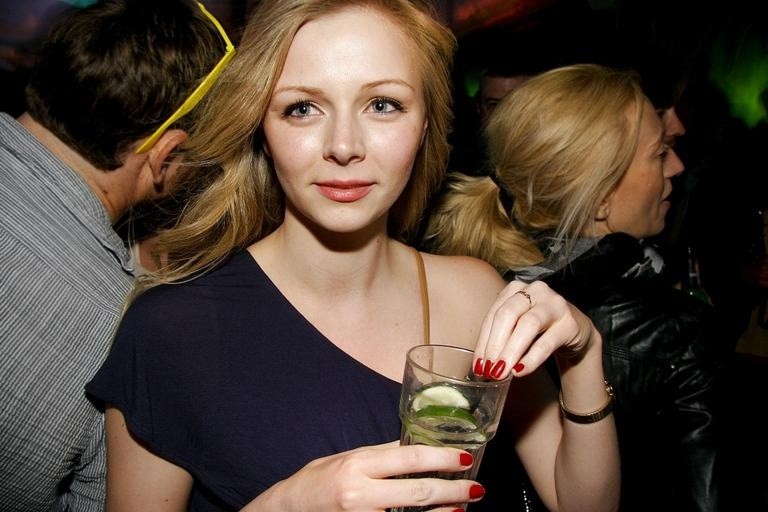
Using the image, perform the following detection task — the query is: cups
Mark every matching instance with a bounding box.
[391,344,513,512]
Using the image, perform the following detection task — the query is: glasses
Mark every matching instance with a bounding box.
[135,0,238,155]
